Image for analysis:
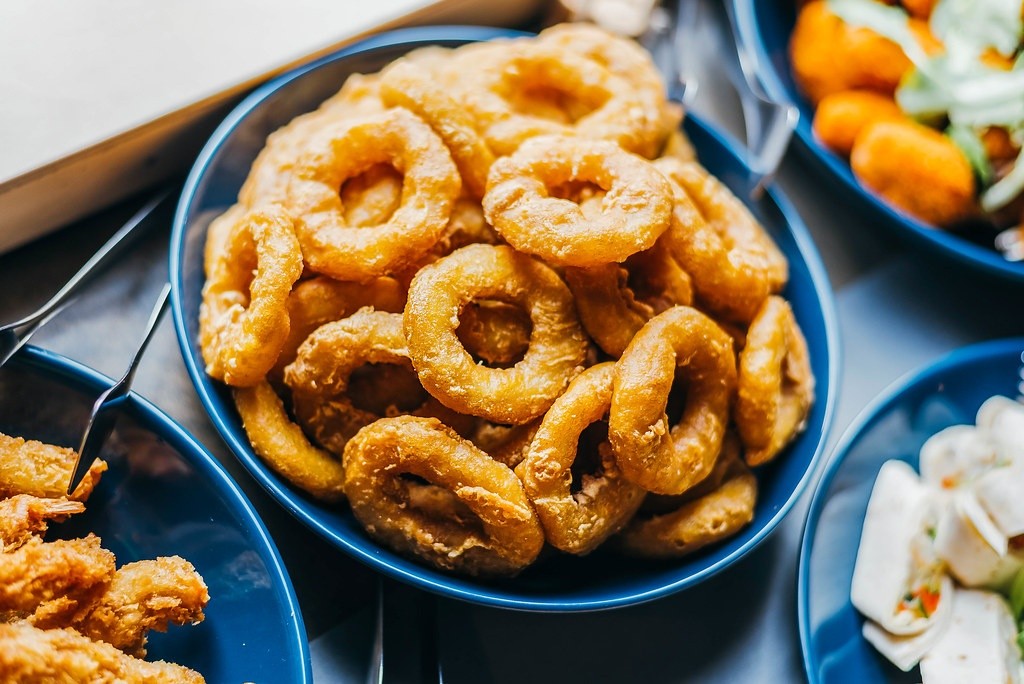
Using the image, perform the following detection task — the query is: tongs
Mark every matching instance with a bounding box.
[647,0,799,205]
[2,195,170,495]
[364,576,445,684]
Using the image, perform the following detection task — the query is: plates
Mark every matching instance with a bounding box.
[170,24,838,612]
[797,337,1024,684]
[0,342,313,684]
[727,0,1024,275]
